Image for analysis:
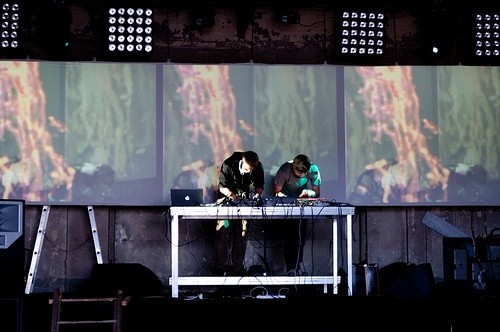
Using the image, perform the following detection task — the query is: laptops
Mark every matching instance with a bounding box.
[170,189,204,206]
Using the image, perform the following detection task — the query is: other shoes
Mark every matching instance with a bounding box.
[287,269,296,276]
[212,268,224,276]
[298,270,308,276]
[236,269,249,276]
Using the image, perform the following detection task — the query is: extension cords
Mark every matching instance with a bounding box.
[256,295,285,299]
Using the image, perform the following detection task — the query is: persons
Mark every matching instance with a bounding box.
[273,154,322,276]
[218,151,267,275]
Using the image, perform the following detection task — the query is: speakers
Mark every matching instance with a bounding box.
[0,199,26,295]
[86,263,165,297]
